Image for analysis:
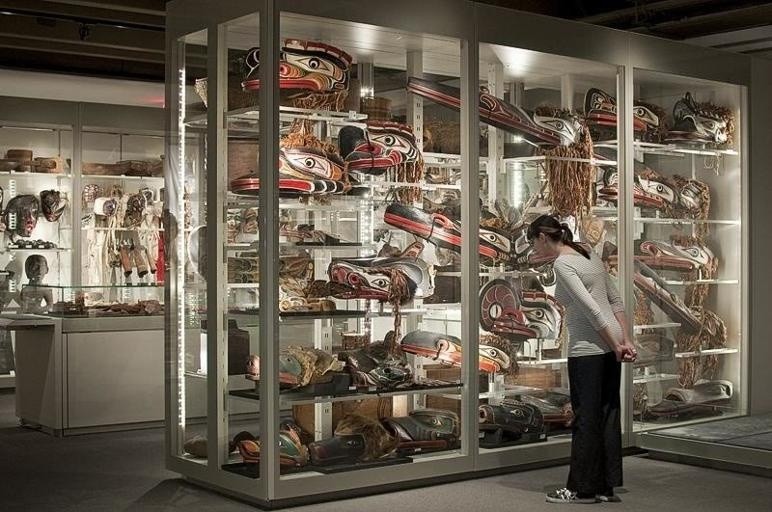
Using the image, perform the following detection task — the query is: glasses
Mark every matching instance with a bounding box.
[529,234,538,246]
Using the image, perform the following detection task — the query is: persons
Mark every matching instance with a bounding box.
[526,214,639,503]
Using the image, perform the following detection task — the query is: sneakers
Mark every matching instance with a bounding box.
[546,487,614,503]
